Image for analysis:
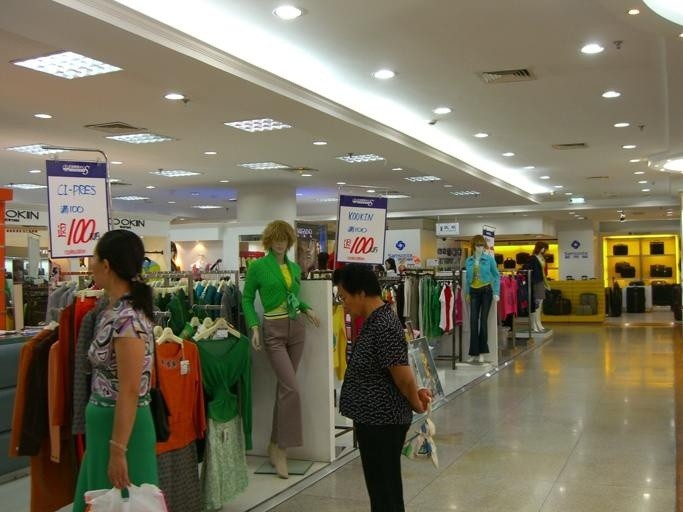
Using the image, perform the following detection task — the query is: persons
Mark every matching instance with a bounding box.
[464,235,499,363]
[331,262,432,511]
[525,241,547,335]
[385,258,396,273]
[374,264,383,272]
[241,220,320,478]
[69,229,160,512]
[397,265,404,273]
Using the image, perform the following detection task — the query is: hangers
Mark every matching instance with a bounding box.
[376,273,460,288]
[41,270,240,346]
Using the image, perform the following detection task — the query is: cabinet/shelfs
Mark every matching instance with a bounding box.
[604,235,677,288]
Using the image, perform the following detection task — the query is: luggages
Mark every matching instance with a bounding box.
[627,287,645,314]
[605,276,622,317]
[652,280,682,320]
[542,288,572,315]
[576,293,597,315]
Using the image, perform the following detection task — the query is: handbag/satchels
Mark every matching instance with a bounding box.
[542,254,554,263]
[613,245,628,254]
[650,241,664,254]
[150,386,172,442]
[495,253,530,268]
[629,280,644,286]
[650,264,672,277]
[615,262,635,277]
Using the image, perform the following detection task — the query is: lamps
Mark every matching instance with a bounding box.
[616,207,626,224]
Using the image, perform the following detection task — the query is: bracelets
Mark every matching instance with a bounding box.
[416,387,428,393]
[109,440,128,451]
